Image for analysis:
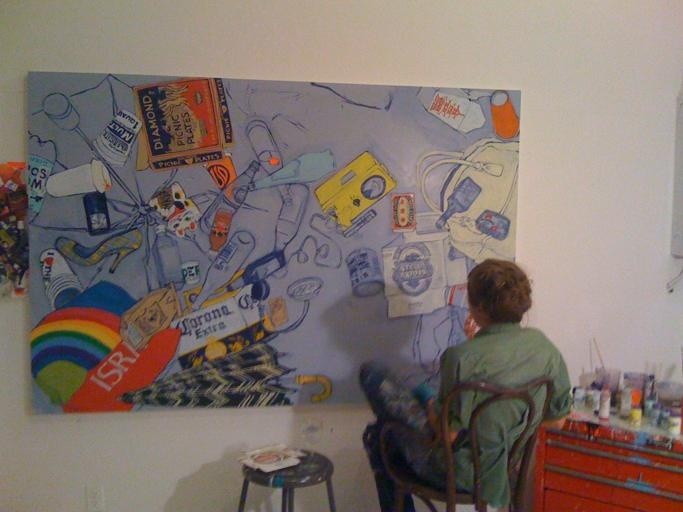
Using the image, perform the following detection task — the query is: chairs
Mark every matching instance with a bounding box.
[376,376,554,512]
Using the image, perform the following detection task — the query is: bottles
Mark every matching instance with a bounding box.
[648,402,682,435]
[208,208,233,257]
[26,135,57,225]
[435,177,482,229]
[447,310,467,347]
[244,119,293,208]
[190,231,256,310]
[248,148,336,192]
[620,387,632,419]
[227,250,285,292]
[151,225,183,291]
[629,402,642,433]
[198,160,260,236]
[274,184,309,252]
[490,90,519,139]
[598,384,610,422]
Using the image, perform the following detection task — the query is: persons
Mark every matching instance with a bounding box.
[357,258,571,511]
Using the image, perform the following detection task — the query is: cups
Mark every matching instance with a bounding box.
[39,248,81,312]
[182,262,200,285]
[92,109,142,169]
[45,160,110,197]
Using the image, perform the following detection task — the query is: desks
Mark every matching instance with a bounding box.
[533,401,682,512]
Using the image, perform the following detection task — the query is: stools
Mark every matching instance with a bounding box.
[236,447,337,512]
